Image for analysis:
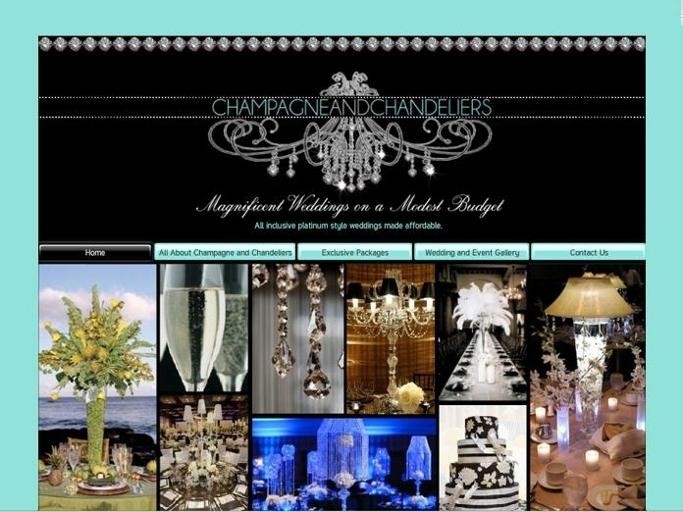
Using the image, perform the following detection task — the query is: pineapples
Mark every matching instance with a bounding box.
[45,445,68,486]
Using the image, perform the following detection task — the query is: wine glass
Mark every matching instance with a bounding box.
[163,264,227,392]
[609,372,625,404]
[57,440,134,482]
[213,264,249,392]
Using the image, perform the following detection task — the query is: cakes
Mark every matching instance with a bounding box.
[440,416,520,512]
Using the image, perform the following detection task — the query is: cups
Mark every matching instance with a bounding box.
[544,462,568,486]
[621,457,644,480]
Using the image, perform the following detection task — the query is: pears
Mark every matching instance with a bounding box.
[146,459,157,474]
[39,459,45,473]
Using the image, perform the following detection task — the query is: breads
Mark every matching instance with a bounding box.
[603,423,635,440]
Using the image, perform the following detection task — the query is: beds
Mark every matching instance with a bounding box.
[530,381,645,510]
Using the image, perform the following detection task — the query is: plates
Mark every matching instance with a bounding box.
[613,467,645,485]
[444,335,527,393]
[530,429,558,444]
[586,483,628,510]
[537,469,572,490]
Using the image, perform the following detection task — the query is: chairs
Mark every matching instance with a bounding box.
[437,330,526,402]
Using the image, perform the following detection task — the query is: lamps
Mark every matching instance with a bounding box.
[344,271,436,414]
[544,278,635,428]
